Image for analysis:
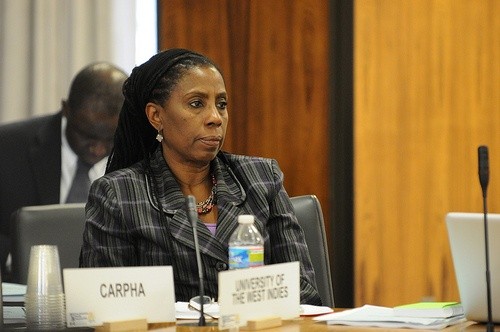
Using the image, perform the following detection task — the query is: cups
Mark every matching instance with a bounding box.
[24,244,67,332]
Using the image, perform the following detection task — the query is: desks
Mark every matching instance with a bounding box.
[93,306,500,332]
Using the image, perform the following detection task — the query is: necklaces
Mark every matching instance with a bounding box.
[196,173,218,215]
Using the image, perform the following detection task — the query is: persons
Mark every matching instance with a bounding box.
[78,48,322,307]
[0,61,128,284]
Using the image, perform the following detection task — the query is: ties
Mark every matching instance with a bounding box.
[65,156,94,203]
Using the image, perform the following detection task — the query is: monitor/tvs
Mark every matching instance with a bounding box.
[446,211,500,323]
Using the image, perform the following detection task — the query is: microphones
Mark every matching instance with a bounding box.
[184,195,206,326]
[477,145,495,331]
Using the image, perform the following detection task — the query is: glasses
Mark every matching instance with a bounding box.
[188,296,218,321]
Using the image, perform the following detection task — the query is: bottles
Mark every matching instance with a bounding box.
[227,215,265,271]
